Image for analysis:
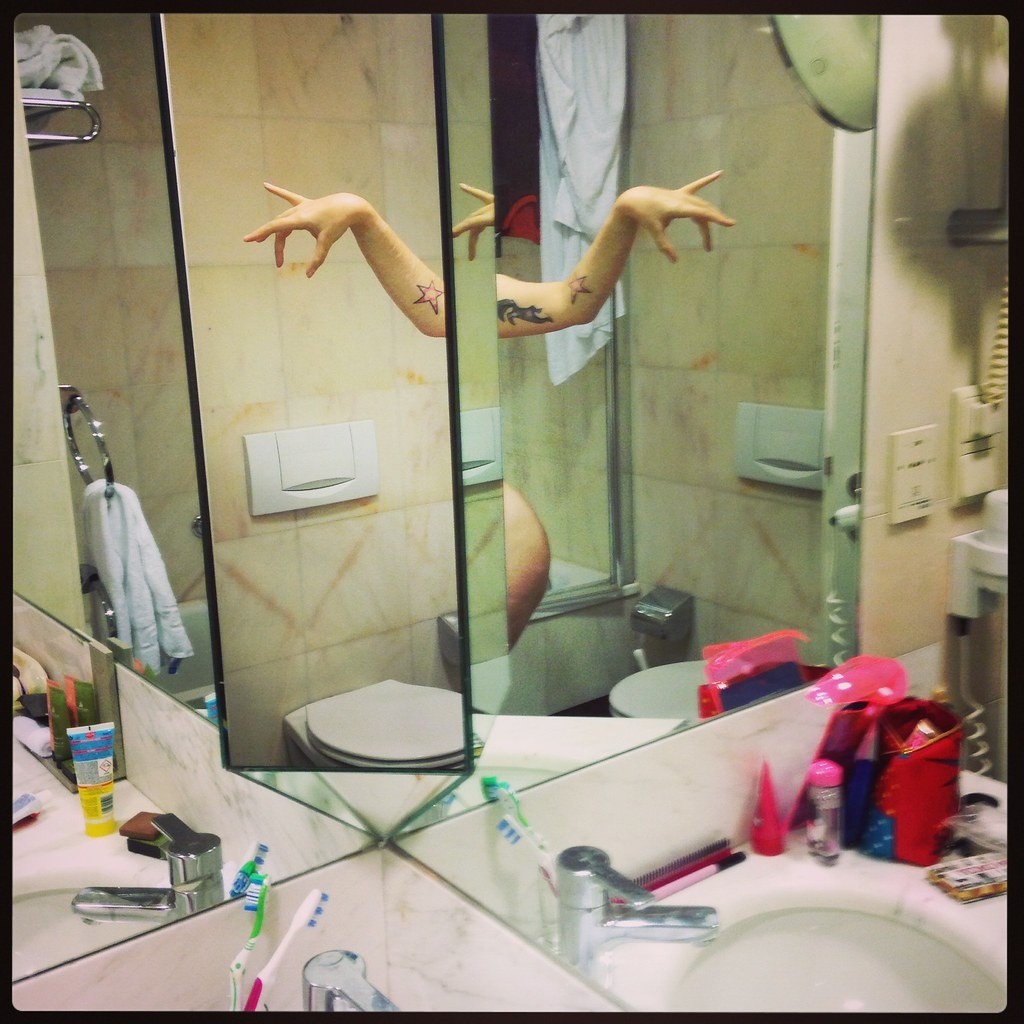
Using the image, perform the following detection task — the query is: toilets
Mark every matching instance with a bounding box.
[280,678,468,775]
[606,658,708,728]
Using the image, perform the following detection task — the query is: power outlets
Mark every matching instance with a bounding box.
[460,406,504,488]
[735,399,826,492]
[242,420,381,519]
[951,383,1003,510]
[889,423,939,528]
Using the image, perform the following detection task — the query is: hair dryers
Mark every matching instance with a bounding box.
[977,489,1008,598]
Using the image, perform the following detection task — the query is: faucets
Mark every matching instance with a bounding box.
[69,813,224,926]
[553,846,720,972]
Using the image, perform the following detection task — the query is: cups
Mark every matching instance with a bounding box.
[804,759,842,868]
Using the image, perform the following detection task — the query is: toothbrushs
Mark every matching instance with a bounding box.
[496,781,566,914]
[228,883,268,1011]
[479,776,541,908]
[236,888,329,1011]
[229,843,269,898]
[495,813,579,925]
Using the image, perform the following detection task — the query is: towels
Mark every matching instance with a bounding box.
[530,10,632,390]
[14,24,106,138]
[80,477,194,679]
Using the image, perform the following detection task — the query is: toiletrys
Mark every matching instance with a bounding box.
[45,673,116,785]
[746,754,785,856]
[66,722,119,840]
[803,758,845,865]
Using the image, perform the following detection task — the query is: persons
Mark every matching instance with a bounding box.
[243,179,445,338]
[507,169,739,653]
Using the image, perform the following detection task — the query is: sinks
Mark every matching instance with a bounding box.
[662,893,1007,1012]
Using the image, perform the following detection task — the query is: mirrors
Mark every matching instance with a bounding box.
[153,7,477,782]
[429,7,838,724]
